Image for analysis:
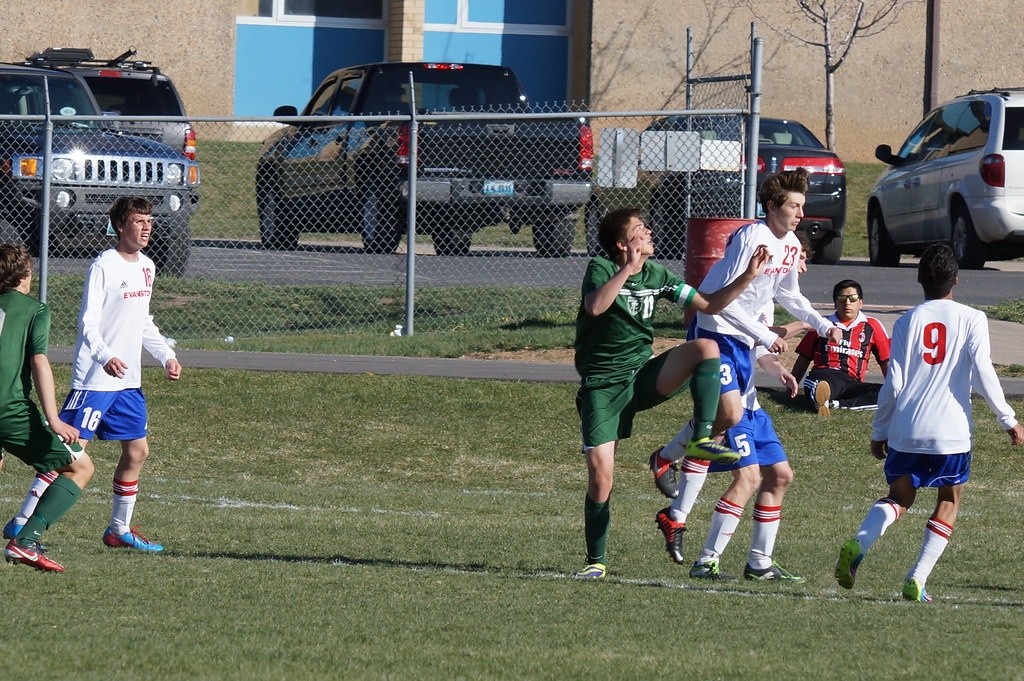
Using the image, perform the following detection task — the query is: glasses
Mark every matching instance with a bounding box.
[837,294,860,302]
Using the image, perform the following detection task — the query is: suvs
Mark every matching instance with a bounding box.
[28,46,197,158]
[0,61,200,277]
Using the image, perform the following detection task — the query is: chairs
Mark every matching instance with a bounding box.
[450,87,478,111]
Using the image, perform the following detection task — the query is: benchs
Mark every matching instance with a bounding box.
[701,131,793,144]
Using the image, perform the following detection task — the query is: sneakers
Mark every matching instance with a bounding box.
[575,563,606,579]
[834,538,863,589]
[688,558,737,581]
[815,381,830,416]
[654,506,688,565]
[648,447,680,499]
[103,525,163,553]
[902,577,932,603]
[3,517,49,553]
[686,436,741,465]
[4,538,64,573]
[743,560,806,584]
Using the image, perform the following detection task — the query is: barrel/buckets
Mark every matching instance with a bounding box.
[684,217,759,330]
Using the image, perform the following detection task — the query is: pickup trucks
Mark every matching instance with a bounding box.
[257,61,595,259]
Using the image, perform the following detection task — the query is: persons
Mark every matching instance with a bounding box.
[0,244,94,573]
[689,230,814,585]
[3,197,182,553]
[835,244,1024,602]
[650,171,843,564]
[786,280,891,416]
[575,211,741,578]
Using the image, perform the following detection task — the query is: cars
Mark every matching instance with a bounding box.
[587,111,846,262]
[866,87,1024,267]
